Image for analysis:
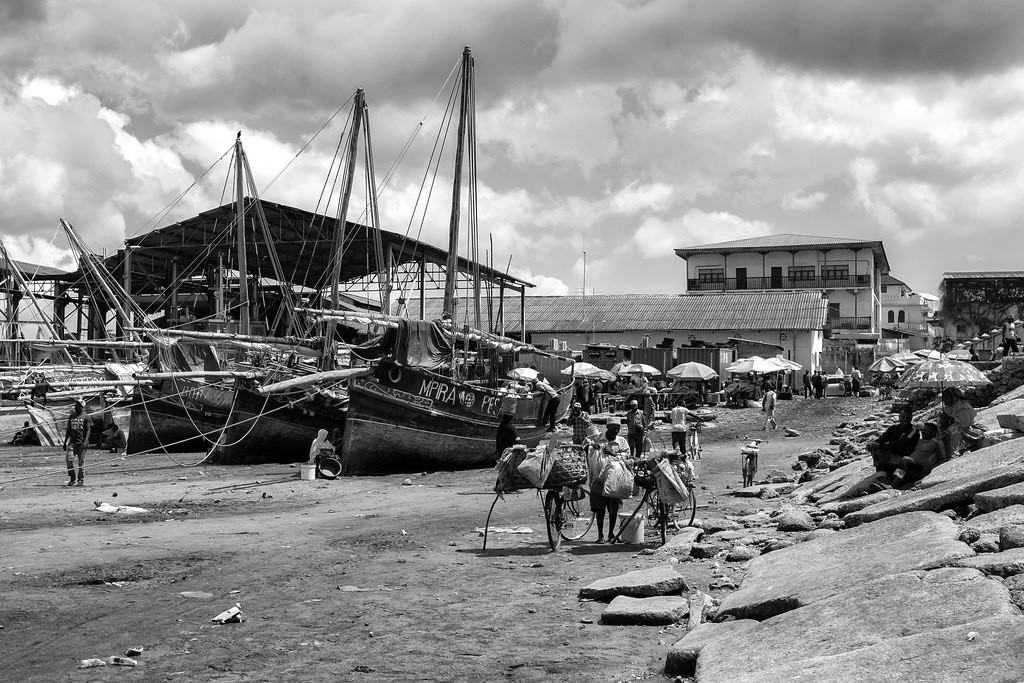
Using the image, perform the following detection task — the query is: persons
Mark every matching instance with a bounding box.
[495,413,523,494]
[567,402,590,445]
[531,378,560,433]
[94,423,126,453]
[803,369,828,399]
[626,399,646,458]
[851,367,861,397]
[755,374,772,401]
[579,378,603,413]
[590,416,630,543]
[835,365,843,374]
[762,387,777,430]
[670,400,704,455]
[63,399,90,486]
[613,377,636,391]
[7,421,35,446]
[724,375,739,388]
[866,387,977,490]
[969,314,1017,361]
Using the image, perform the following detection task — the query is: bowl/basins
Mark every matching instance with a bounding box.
[318,458,342,479]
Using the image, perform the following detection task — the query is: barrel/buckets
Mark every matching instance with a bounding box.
[619,513,645,544]
[301,464,316,481]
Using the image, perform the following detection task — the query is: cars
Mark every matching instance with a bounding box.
[806,375,846,398]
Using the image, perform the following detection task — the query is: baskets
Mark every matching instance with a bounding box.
[689,425,702,433]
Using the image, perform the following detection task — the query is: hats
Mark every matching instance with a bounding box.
[531,378,537,384]
[631,400,638,405]
[606,417,622,425]
[573,403,581,408]
[73,398,85,407]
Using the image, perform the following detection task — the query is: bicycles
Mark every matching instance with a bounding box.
[739,435,772,488]
[538,482,596,552]
[637,455,697,546]
[687,419,709,460]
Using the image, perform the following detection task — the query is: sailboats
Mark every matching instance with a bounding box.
[0,44,586,481]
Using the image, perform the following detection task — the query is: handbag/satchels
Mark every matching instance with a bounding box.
[599,459,635,498]
[519,446,556,489]
[653,457,690,503]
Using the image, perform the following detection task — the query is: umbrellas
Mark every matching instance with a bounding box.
[560,363,719,381]
[867,348,993,386]
[726,355,803,389]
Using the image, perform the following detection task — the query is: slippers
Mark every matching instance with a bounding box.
[596,537,606,544]
[608,536,620,542]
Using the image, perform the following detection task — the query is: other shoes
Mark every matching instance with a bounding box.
[68,481,76,486]
[78,482,84,486]
[878,482,892,490]
[869,483,882,493]
